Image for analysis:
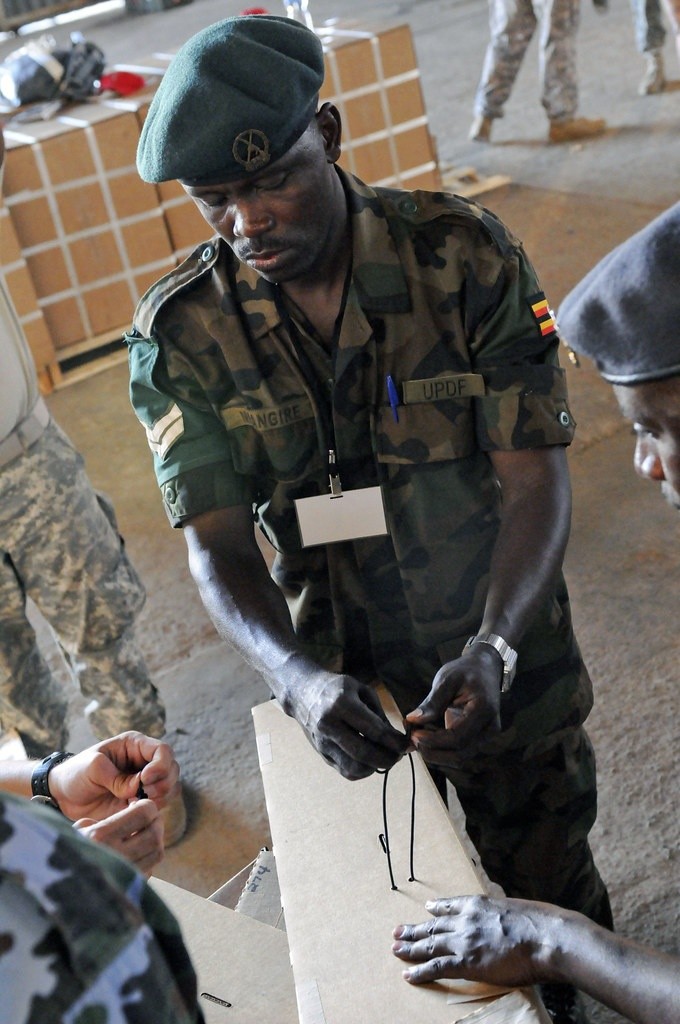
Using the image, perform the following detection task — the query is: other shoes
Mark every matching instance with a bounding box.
[548,119,606,139]
[467,114,495,143]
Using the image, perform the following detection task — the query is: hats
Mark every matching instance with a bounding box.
[135,15,326,187]
[553,201,680,383]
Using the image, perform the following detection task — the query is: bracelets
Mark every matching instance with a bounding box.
[32,751,75,817]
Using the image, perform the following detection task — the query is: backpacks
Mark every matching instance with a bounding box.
[2,41,106,103]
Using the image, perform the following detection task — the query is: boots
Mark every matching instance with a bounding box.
[639,52,664,96]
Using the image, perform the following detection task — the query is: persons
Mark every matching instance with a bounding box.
[392,196,679,1017]
[632,1,678,95]
[123,12,617,1024]
[472,1,608,145]
[0,729,205,883]
[2,262,168,770]
[0,791,209,1022]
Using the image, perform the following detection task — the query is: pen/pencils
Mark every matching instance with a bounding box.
[386,374,399,424]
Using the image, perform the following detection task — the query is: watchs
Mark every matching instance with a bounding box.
[460,633,519,692]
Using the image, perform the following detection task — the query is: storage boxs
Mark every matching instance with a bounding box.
[80,50,217,269]
[0,194,56,373]
[152,17,443,191]
[2,98,179,354]
